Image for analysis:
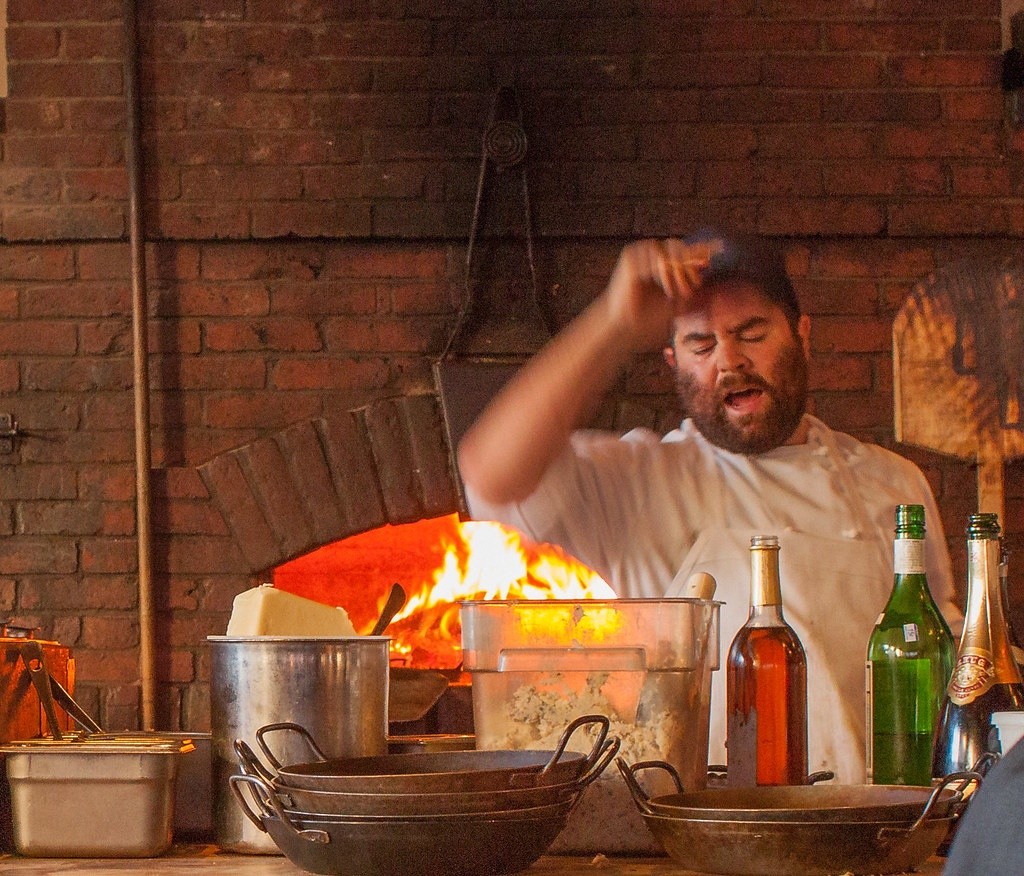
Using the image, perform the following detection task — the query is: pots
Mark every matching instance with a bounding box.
[229,714,1001,876]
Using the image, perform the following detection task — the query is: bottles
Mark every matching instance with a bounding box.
[865,505,956,787]
[726,535,810,787]
[930,513,1024,857]
[997,536,1024,679]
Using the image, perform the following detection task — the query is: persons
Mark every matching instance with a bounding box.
[457,235,968,788]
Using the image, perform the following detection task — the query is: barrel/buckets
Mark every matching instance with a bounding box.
[206,636,392,857]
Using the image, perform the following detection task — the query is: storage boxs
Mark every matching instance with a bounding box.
[459,597,729,858]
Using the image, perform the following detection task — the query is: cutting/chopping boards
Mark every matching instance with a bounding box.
[891,260,1024,539]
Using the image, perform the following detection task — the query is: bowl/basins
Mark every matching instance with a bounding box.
[1,734,213,858]
